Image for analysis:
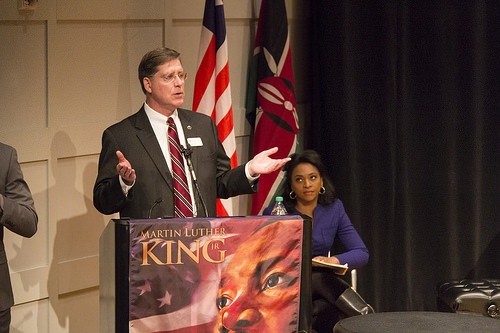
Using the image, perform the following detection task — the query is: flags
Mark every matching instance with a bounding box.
[190,0,239,219]
[243,0,302,215]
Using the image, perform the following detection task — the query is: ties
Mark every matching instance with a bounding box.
[166,117,195,218]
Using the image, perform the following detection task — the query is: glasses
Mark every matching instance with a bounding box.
[147,72,188,83]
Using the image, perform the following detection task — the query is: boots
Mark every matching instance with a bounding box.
[335,286,375,317]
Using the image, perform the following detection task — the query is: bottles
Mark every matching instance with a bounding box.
[271,196,287,216]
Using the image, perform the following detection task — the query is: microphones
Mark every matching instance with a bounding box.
[180,143,209,218]
[147,199,163,218]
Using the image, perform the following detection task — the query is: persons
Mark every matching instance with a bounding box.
[91,47,291,222]
[214,215,303,332]
[261,148,380,332]
[0,139,41,333]
